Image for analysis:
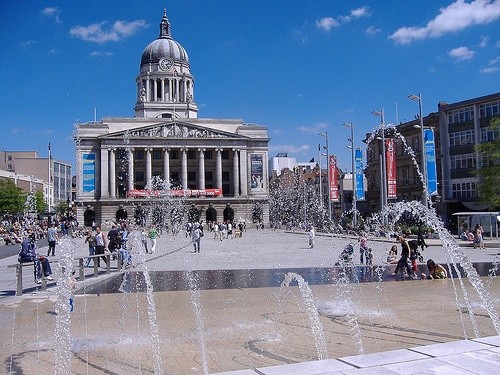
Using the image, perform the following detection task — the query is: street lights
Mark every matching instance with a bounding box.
[318,131,332,221]
[408,93,429,209]
[370,109,389,229]
[343,120,357,226]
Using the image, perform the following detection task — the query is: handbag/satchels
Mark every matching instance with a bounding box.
[462,236,468,241]
[476,239,480,243]
[89,241,96,247]
[200,233,204,237]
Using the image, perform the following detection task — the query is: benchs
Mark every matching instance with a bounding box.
[7,251,121,296]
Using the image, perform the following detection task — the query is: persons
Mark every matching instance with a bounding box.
[473,224,485,249]
[379,225,411,238]
[150,221,182,234]
[208,220,246,240]
[107,220,135,267]
[140,227,161,253]
[330,222,366,236]
[257,220,264,230]
[84,226,108,267]
[387,236,447,280]
[47,223,59,256]
[461,231,474,241]
[0,220,79,245]
[17,232,53,284]
[425,223,435,239]
[308,226,315,248]
[335,237,373,265]
[186,220,203,253]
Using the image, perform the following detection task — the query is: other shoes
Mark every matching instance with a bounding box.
[44,276,54,280]
[47,253,49,256]
[479,247,486,249]
[151,249,153,254]
[391,273,396,275]
[35,280,42,284]
[52,253,56,256]
[413,271,417,277]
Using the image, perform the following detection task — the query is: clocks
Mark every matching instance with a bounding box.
[159,57,174,71]
